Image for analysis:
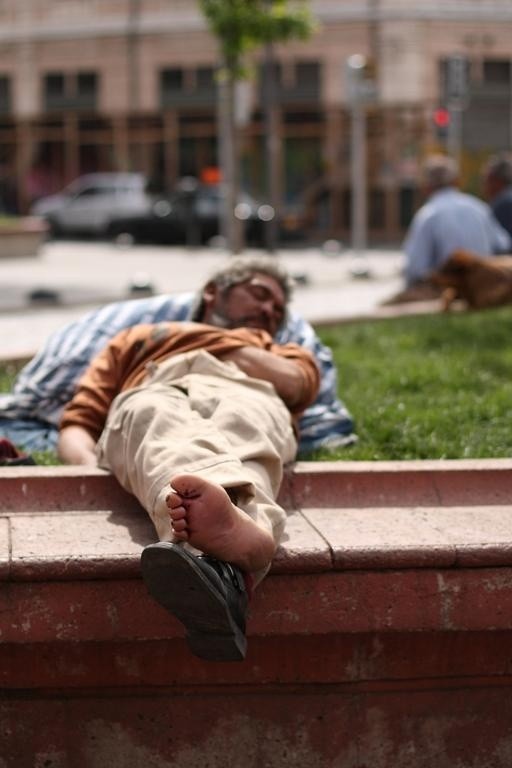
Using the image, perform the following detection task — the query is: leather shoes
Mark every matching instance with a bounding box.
[138,541,249,663]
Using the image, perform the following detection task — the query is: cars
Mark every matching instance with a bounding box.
[28,171,266,248]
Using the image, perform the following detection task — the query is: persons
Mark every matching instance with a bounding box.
[481,158,511,227]
[51,258,321,668]
[367,152,511,308]
